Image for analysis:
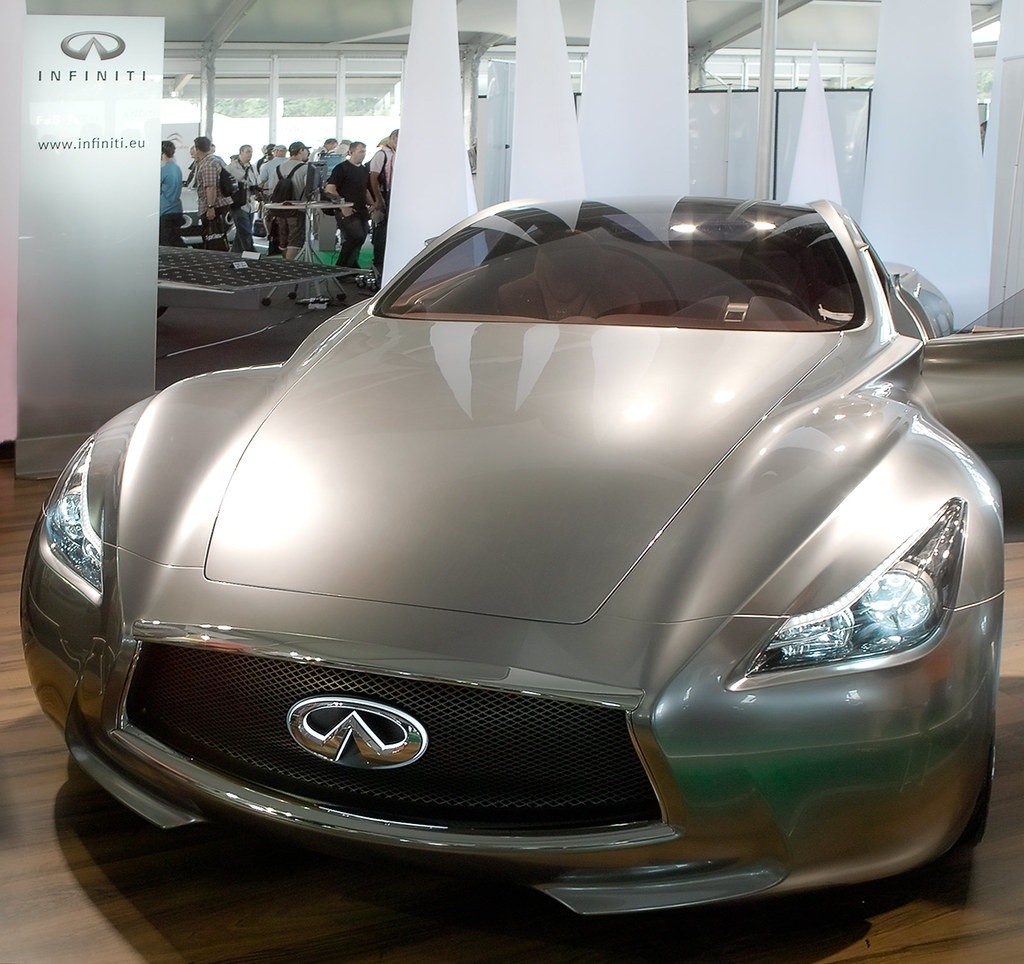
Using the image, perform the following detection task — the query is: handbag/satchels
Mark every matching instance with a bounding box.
[220,167,238,196]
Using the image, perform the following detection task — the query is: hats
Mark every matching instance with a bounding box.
[271,145,287,153]
[289,141,312,153]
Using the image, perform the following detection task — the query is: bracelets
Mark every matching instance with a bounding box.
[208,205,214,208]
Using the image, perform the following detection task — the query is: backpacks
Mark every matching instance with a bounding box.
[269,163,306,217]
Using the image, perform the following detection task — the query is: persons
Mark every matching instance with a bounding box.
[159,128,398,270]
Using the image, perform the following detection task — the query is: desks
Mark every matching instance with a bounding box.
[261,200,354,306]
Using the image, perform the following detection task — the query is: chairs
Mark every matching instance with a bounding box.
[498,230,641,320]
[697,230,853,324]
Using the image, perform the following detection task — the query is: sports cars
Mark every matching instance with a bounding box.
[18,191,1024,930]
